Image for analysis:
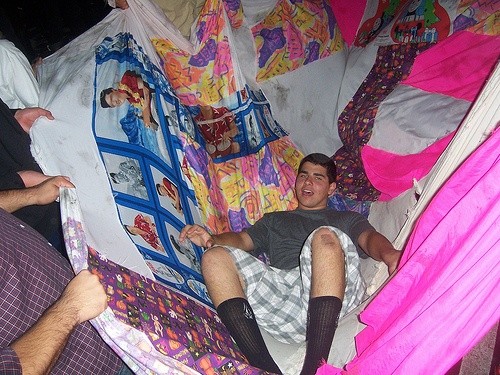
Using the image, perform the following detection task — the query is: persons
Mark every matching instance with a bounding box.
[0,176,136,375]
[179,153,403,375]
[0,0,131,262]
